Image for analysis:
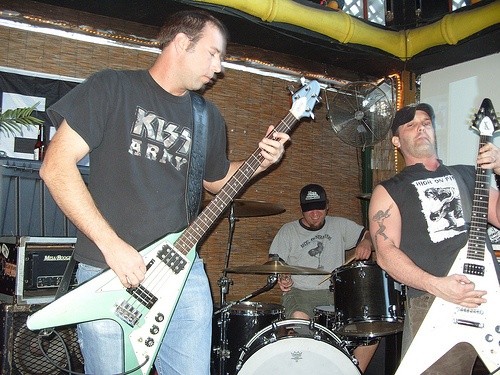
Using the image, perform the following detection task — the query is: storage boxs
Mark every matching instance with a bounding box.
[0,235,77,306]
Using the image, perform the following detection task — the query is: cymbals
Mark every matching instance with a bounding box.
[221,259,331,276]
[198,193,287,219]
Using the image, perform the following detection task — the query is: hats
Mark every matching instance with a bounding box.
[300,184,329,213]
[392,102,434,134]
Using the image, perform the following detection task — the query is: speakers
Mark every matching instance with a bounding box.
[0,303,84,375]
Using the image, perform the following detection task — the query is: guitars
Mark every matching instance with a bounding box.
[393,94,500,375]
[26,75,324,374]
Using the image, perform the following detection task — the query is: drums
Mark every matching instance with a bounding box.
[331,261,405,338]
[315,305,381,347]
[232,318,365,375]
[220,297,286,374]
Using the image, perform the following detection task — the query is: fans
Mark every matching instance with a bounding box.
[324,77,395,151]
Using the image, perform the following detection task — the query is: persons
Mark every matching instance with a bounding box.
[38,12,290,375]
[368,104,500,375]
[268,183,380,372]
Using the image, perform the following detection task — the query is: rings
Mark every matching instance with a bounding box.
[491,155,496,163]
